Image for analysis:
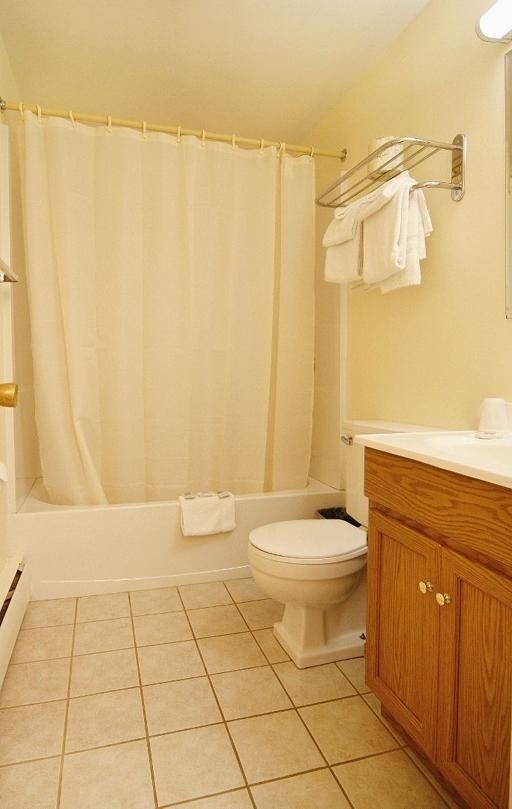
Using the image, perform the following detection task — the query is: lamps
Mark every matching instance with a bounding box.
[470,0,511,50]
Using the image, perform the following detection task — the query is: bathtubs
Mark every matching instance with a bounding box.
[15,476,343,598]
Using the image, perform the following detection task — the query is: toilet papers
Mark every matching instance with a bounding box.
[480,399,506,433]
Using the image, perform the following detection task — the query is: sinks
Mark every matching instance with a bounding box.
[393,433,512,476]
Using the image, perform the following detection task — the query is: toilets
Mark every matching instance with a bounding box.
[246,419,449,670]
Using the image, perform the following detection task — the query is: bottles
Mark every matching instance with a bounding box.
[477,397,507,438]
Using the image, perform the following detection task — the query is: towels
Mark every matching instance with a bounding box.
[178,490,239,540]
[320,167,439,296]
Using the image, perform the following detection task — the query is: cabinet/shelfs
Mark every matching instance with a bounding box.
[0,119,35,688]
[355,502,511,808]
